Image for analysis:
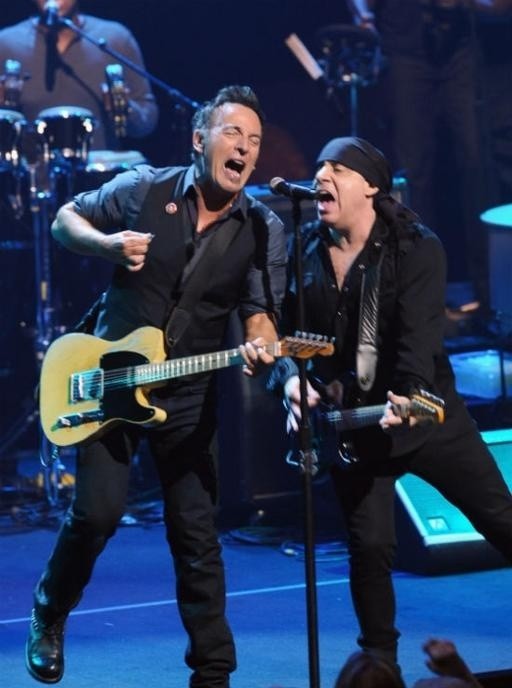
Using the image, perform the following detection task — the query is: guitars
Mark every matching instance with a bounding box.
[39,325,336,450]
[276,390,448,477]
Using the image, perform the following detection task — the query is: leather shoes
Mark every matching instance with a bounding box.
[25,609,66,685]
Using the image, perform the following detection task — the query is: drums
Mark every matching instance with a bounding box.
[0,157,34,248]
[35,105,93,170]
[480,205,512,348]
[0,109,25,159]
[53,151,147,206]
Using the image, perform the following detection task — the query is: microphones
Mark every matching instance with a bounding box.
[268,177,320,200]
[42,0,59,26]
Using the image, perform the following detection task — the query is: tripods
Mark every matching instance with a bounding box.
[1,173,163,525]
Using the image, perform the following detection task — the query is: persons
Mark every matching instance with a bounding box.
[2,0,159,474]
[329,638,481,688]
[259,135,512,662]
[412,676,471,687]
[24,85,279,686]
[352,2,508,309]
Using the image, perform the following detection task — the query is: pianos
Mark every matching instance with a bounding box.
[244,178,408,211]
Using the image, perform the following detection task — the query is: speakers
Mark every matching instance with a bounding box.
[361,427,512,566]
